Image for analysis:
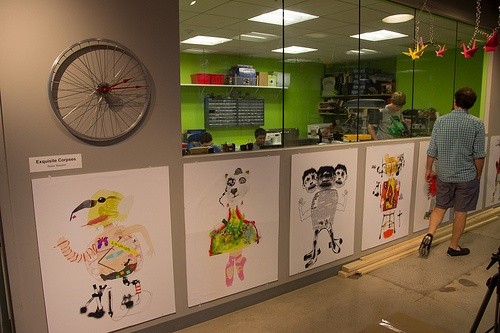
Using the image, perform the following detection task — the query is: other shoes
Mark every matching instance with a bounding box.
[418,233,433,257]
[447,245,470,257]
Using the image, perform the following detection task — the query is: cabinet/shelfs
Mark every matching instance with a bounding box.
[317,94,392,114]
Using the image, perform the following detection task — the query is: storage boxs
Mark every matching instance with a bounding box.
[192,65,276,87]
[342,133,371,142]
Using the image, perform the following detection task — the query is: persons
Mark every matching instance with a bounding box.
[251,128,273,150]
[418,86,486,259]
[196,132,221,153]
[368,91,406,141]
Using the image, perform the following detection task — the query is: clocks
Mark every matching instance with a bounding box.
[48,37,153,144]
